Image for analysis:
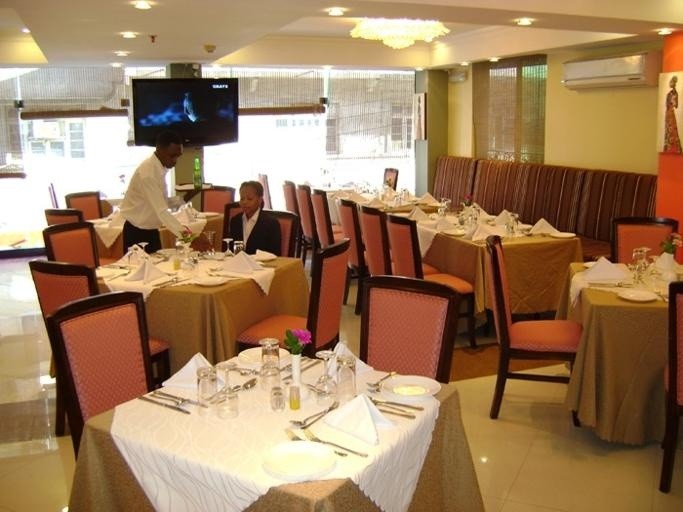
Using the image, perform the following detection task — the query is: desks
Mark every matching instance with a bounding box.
[94,250,302,336]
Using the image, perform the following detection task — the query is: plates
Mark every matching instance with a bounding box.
[381,374,440,398]
[584,261,616,269]
[617,289,656,302]
[550,232,575,238]
[262,439,337,478]
[198,211,220,218]
[237,344,288,370]
[92,267,114,281]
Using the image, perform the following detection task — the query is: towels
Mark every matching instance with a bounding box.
[581,256,626,283]
[320,393,398,446]
[160,351,229,394]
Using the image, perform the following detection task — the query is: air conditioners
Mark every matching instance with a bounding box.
[558,47,661,91]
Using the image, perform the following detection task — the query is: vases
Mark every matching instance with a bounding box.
[289,352,304,392]
[653,252,677,275]
[180,243,195,271]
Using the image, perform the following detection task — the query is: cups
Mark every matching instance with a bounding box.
[269,381,286,411]
[418,197,533,240]
[352,177,410,208]
[196,365,218,404]
[214,361,239,417]
[336,354,357,401]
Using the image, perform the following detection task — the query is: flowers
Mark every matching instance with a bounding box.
[658,230,682,253]
[282,326,314,353]
[178,228,193,242]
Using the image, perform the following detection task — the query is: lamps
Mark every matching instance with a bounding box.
[133,1,151,9]
[656,29,672,35]
[517,17,533,28]
[109,29,139,70]
[326,6,344,16]
[348,15,450,51]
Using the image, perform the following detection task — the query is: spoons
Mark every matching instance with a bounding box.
[289,402,339,427]
[367,396,423,421]
[209,377,258,403]
[365,371,392,392]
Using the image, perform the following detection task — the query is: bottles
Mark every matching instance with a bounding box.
[288,385,301,410]
[192,155,201,189]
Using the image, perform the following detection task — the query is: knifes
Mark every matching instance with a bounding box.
[280,356,319,381]
[158,391,207,407]
[137,395,191,414]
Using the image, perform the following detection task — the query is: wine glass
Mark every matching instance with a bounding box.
[313,349,336,405]
[628,247,651,283]
[122,231,244,287]
[259,336,280,394]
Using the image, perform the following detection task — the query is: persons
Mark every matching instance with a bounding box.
[182,91,198,123]
[120,129,214,256]
[226,180,282,256]
[664,76,681,153]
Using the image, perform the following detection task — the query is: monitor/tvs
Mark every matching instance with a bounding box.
[131,78,238,146]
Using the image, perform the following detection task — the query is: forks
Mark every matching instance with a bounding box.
[283,427,346,457]
[302,428,367,456]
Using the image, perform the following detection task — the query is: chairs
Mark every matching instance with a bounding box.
[354,273,462,385]
[200,153,581,354]
[483,235,583,428]
[42,289,156,465]
[63,190,102,220]
[608,215,678,266]
[656,281,681,494]
[43,222,100,267]
[43,208,84,225]
[28,259,98,378]
